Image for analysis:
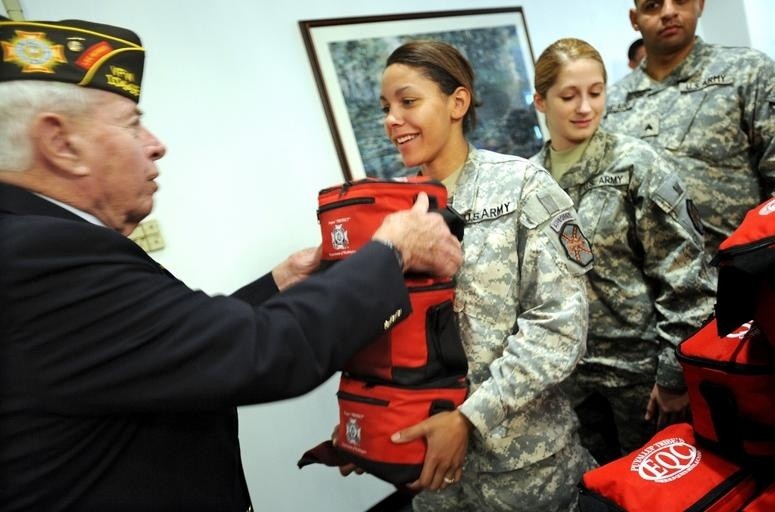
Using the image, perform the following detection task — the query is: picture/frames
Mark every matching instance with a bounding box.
[298,6,551,184]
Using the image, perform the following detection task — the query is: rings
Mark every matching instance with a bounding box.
[444,477,454,484]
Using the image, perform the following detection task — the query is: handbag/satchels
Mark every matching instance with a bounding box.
[333,376,470,484]
[582,422,773,511]
[319,178,463,262]
[341,275,469,385]
[676,195,775,484]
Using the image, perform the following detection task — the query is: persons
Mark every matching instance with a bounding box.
[0,15,465,512]
[330,40,596,512]
[600,0,775,256]
[627,38,647,70]
[526,37,719,463]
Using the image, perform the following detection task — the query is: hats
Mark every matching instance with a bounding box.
[1,16,144,102]
